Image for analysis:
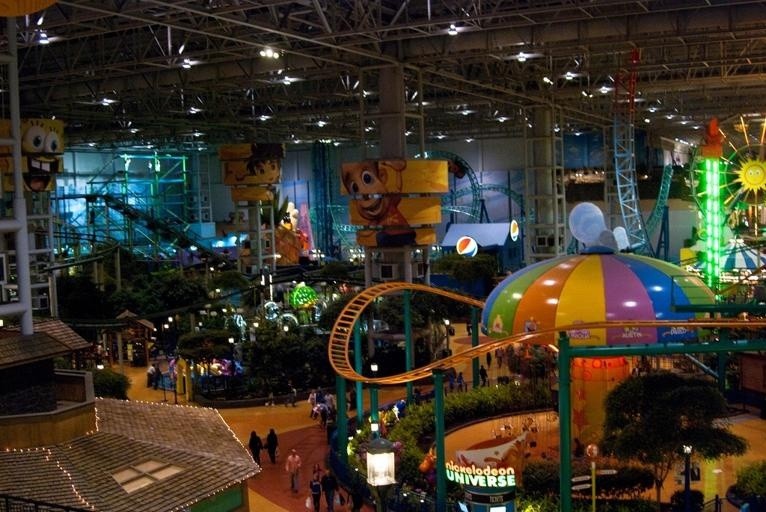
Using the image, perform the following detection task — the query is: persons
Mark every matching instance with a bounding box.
[449,372,465,392]
[147,364,164,390]
[80,347,104,369]
[691,462,700,480]
[308,387,337,427]
[479,344,514,386]
[348,471,364,510]
[414,386,422,405]
[284,380,296,406]
[249,431,263,466]
[265,428,279,464]
[309,462,340,512]
[466,319,472,336]
[265,386,275,406]
[286,448,301,492]
[150,341,158,360]
[346,386,357,411]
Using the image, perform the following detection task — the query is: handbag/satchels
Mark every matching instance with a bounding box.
[305,497,312,511]
[275,447,279,456]
[261,451,269,463]
[263,443,268,449]
[336,493,345,506]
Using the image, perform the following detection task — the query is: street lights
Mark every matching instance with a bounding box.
[362,437,399,512]
[684,440,693,490]
